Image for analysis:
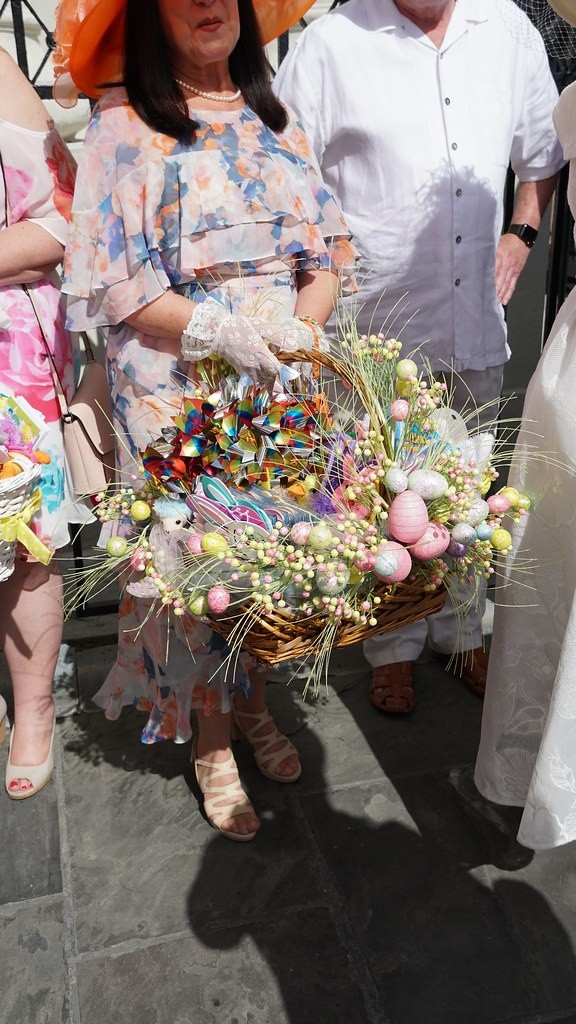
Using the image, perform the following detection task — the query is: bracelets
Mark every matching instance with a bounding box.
[296,315,324,329]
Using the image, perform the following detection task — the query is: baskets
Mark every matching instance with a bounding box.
[123,349,450,665]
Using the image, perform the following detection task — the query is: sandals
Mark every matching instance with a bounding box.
[367,661,417,713]
[448,647,490,696]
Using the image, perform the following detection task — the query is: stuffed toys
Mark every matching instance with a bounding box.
[152,496,192,535]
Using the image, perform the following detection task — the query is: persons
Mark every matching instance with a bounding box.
[272,0,568,712]
[0,43,79,798]
[474,0,576,851]
[51,0,351,840]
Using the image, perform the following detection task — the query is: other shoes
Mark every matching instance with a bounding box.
[5,695,57,800]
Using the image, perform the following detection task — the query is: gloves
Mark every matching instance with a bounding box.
[247,315,331,379]
[179,295,282,385]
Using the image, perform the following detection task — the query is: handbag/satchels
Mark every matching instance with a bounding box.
[59,359,116,495]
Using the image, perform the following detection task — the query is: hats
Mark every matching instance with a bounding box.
[54,0,317,108]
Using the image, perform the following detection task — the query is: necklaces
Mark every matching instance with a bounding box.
[173,76,242,102]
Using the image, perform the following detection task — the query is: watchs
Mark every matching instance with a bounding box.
[508,224,538,246]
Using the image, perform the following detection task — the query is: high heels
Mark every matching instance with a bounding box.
[228,691,302,783]
[189,730,261,841]
